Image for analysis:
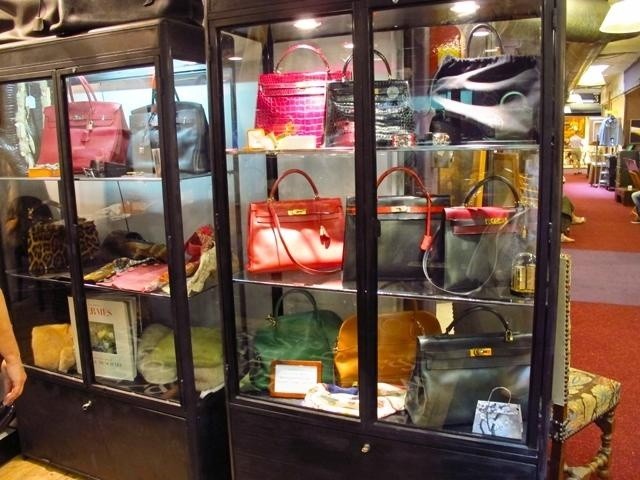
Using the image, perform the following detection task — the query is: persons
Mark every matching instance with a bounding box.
[0,288,27,407]
[570,130,584,175]
[630,190,640,224]
[560,175,587,243]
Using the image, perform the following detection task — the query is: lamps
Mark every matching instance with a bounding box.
[598,0,640,34]
[566,88,583,103]
[576,65,609,85]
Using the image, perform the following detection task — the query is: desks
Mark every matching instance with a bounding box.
[587,162,609,187]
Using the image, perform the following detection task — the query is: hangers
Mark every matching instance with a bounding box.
[610,113,616,120]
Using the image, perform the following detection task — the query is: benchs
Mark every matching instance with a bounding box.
[615,150,640,205]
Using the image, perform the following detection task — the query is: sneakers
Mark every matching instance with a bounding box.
[561,234,576,243]
[570,216,585,225]
[629,216,640,224]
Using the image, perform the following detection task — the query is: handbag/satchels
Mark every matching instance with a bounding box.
[250,288,344,392]
[439,174,539,291]
[405,304,533,427]
[253,42,352,147]
[129,72,211,174]
[244,169,346,275]
[338,165,451,285]
[25,200,102,275]
[326,47,415,148]
[427,19,540,141]
[332,310,442,386]
[470,386,525,440]
[37,76,132,175]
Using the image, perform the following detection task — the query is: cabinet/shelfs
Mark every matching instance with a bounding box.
[0,18,246,480]
[200,0,566,480]
[598,154,617,189]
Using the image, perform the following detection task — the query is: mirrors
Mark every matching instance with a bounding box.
[629,119,640,145]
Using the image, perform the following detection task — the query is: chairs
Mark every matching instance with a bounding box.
[549,255,621,479]
